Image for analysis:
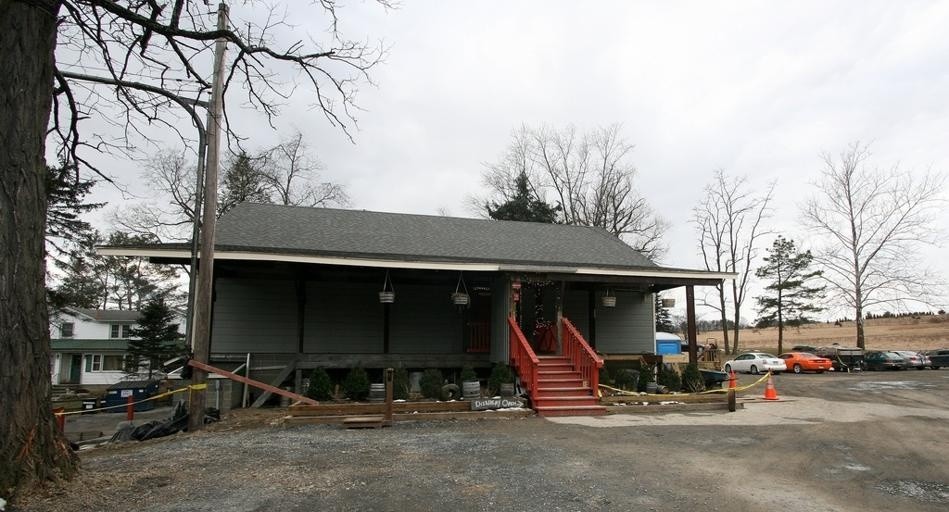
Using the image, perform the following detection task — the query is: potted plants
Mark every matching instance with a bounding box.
[460,370,480,401]
[492,364,515,396]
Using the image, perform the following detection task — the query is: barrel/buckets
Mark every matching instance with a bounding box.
[645,381,670,394]
[500,383,515,398]
[452,274,469,305]
[462,380,481,401]
[368,383,385,404]
[279,385,294,406]
[408,371,425,393]
[661,288,676,307]
[601,286,616,308]
[378,269,395,304]
[615,368,640,392]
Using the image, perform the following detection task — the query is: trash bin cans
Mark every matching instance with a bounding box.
[81,398,96,415]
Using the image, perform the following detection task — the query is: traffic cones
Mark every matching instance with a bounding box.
[727,369,737,389]
[759,366,782,400]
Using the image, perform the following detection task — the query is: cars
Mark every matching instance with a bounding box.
[863,350,906,373]
[725,352,787,374]
[922,349,949,369]
[891,350,932,370]
[779,352,831,376]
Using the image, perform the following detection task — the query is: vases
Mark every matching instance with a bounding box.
[452,293,468,304]
[378,292,394,303]
[602,297,616,307]
